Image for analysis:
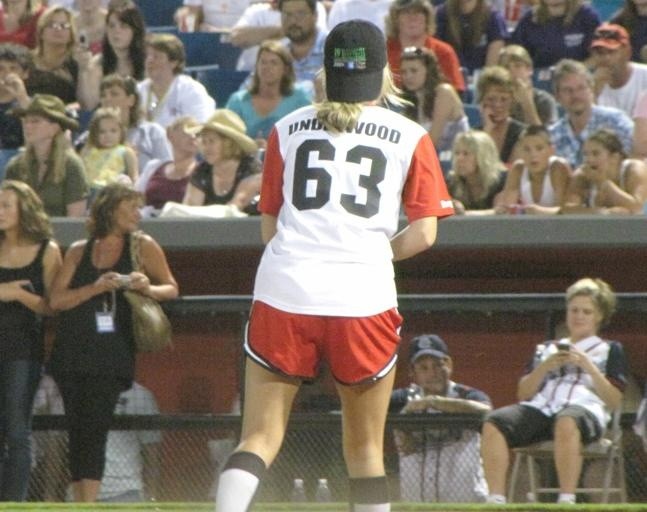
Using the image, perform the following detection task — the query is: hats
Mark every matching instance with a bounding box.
[405,333,452,366]
[6,93,80,129]
[322,18,391,105]
[181,107,259,155]
[588,22,631,51]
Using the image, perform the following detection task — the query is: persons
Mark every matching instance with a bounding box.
[24,5,80,114]
[73,1,147,114]
[546,59,635,170]
[446,129,509,211]
[70,0,108,47]
[483,277,627,507]
[49,381,170,503]
[327,0,390,41]
[400,46,470,179]
[472,64,528,163]
[608,0,647,61]
[494,124,571,216]
[509,0,602,68]
[0,41,37,150]
[561,128,647,216]
[139,34,216,127]
[590,23,647,115]
[383,0,465,92]
[173,0,251,33]
[227,0,328,47]
[216,17,456,512]
[182,109,262,216]
[49,183,179,502]
[433,0,509,75]
[0,180,63,503]
[383,333,492,505]
[495,44,559,127]
[132,116,200,219]
[225,39,312,149]
[75,72,173,174]
[79,107,138,193]
[0,0,48,43]
[237,0,330,91]
[4,92,91,218]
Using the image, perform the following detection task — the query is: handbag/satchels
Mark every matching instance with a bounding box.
[123,226,174,356]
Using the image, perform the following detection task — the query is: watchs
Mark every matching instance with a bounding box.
[434,395,440,410]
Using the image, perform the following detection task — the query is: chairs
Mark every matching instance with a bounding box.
[218,42,242,69]
[0,150,20,180]
[145,26,177,34]
[508,400,627,504]
[593,0,626,22]
[176,31,220,67]
[196,71,250,108]
[134,0,182,26]
[463,105,485,131]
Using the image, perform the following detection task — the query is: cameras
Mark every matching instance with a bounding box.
[113,275,134,289]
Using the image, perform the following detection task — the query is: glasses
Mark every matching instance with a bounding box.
[49,21,72,31]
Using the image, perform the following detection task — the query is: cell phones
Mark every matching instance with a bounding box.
[75,28,88,49]
[556,345,570,354]
